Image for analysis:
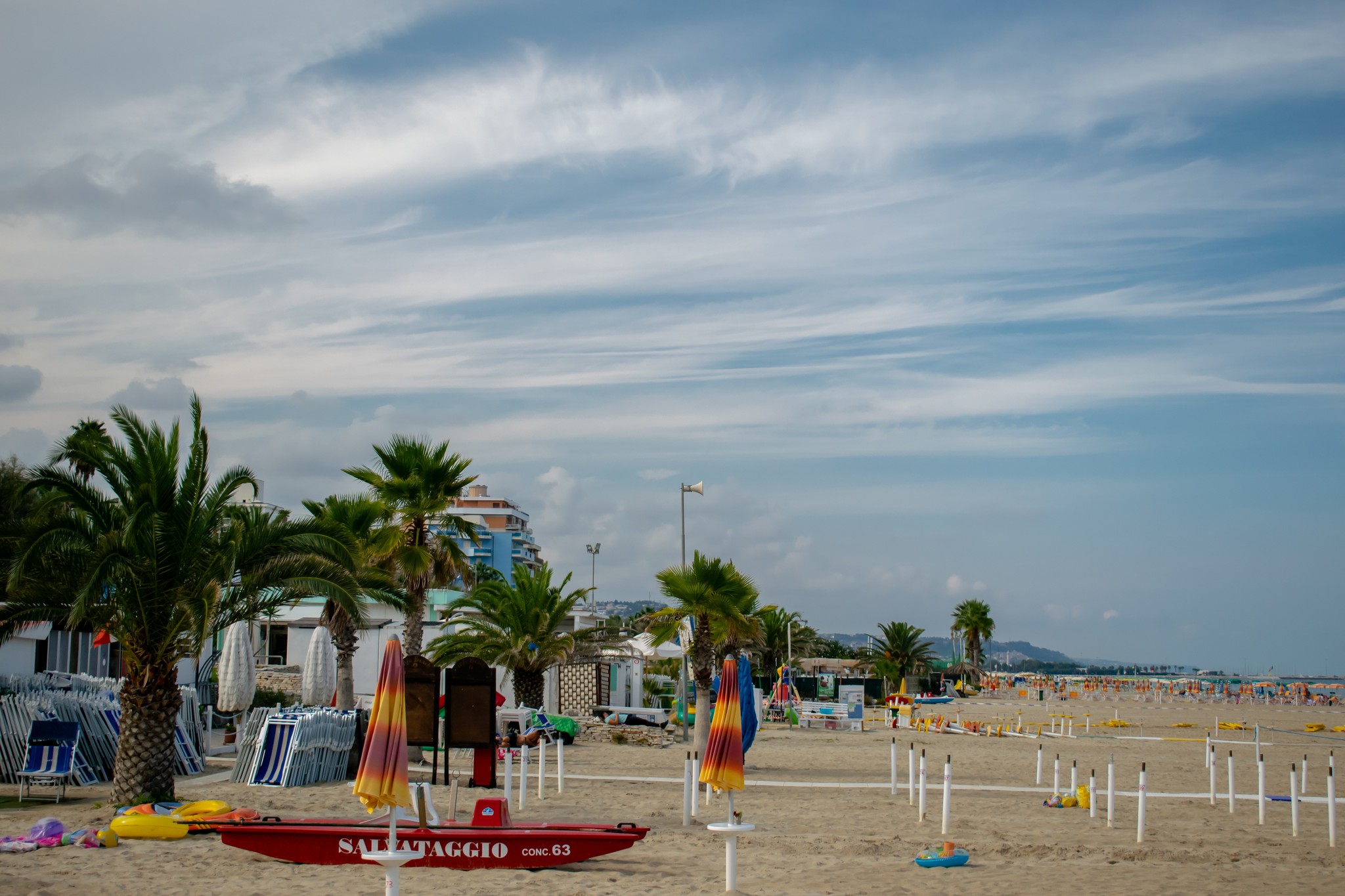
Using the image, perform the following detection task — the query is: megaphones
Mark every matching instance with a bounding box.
[683,481,704,495]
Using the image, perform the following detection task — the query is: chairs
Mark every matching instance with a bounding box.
[2,665,209,788]
[522,708,556,742]
[228,703,362,787]
[16,718,84,805]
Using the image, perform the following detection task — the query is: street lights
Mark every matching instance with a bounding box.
[585,543,601,612]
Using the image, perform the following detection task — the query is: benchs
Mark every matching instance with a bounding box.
[797,716,865,733]
[791,700,848,727]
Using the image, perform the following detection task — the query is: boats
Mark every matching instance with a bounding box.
[914,697,955,703]
[173,816,651,871]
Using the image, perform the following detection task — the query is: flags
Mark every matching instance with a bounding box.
[1268,666,1273,672]
[93,607,125,648]
[1151,668,1154,671]
[1132,663,1136,669]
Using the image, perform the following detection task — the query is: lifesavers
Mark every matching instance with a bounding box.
[171,800,231,817]
[110,815,188,838]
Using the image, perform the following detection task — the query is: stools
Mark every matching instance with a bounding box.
[498,708,534,735]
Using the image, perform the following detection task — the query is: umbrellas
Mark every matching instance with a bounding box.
[1326,683,1345,694]
[898,676,908,696]
[698,653,745,825]
[352,633,413,852]
[602,632,683,699]
[944,661,988,694]
[1309,682,1330,695]
[980,672,1311,707]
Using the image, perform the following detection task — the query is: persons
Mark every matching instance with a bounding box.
[940,678,948,697]
[598,710,669,729]
[498,730,550,748]
[1313,692,1336,707]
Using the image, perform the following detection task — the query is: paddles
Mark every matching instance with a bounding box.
[177,821,651,832]
